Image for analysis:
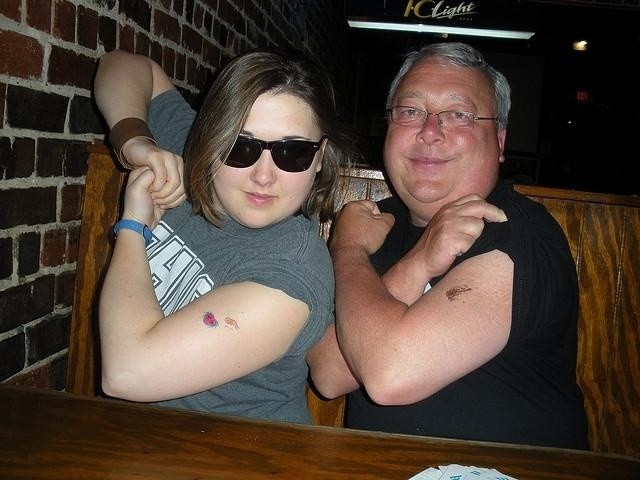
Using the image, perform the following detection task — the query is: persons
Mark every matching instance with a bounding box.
[92,39,337,423]
[303,41,590,450]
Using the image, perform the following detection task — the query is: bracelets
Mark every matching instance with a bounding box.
[113,218,154,248]
[108,116,159,170]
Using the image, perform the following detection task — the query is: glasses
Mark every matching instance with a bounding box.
[384,105,497,132]
[220,135,326,173]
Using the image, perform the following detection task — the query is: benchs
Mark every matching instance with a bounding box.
[68,143,640,460]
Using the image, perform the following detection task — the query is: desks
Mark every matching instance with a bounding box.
[0,384,640,480]
